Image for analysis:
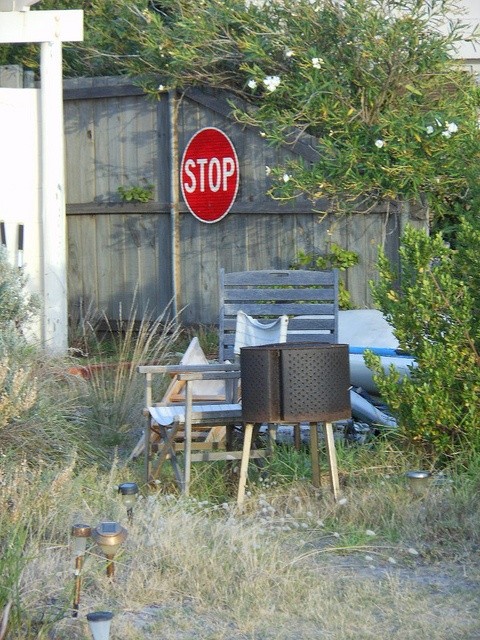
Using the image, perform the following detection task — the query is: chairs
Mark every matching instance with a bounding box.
[138,266,344,501]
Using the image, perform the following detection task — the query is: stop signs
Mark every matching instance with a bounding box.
[180,126,240,224]
